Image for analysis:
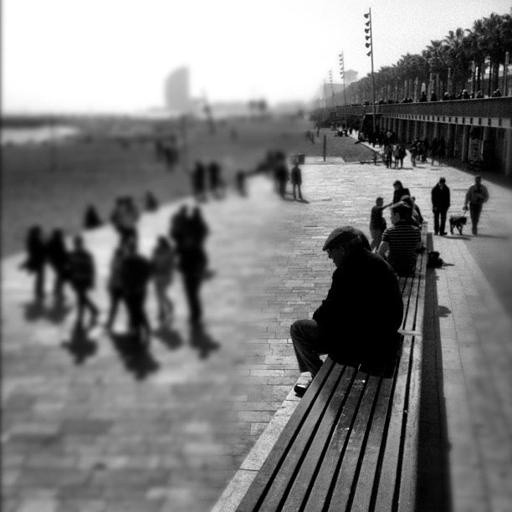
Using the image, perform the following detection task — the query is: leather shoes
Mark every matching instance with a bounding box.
[433,230,449,236]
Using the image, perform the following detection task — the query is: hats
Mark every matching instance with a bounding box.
[322,224,356,251]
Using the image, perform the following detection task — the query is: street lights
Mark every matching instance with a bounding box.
[328,70,336,109]
[363,7,378,140]
[338,51,347,106]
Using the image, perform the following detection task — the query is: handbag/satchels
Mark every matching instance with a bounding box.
[347,326,404,379]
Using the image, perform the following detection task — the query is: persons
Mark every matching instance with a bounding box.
[431,176,450,235]
[290,162,303,200]
[106,195,211,331]
[289,226,404,397]
[464,176,489,234]
[24,225,98,333]
[357,89,502,170]
[191,160,245,193]
[367,175,423,279]
[275,161,289,198]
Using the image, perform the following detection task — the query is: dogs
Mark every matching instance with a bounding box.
[448,216,467,235]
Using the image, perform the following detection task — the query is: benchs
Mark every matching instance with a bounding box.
[234,219,429,512]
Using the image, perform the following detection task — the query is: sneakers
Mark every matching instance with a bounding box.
[294,381,313,395]
